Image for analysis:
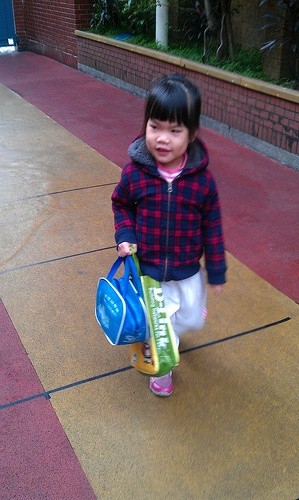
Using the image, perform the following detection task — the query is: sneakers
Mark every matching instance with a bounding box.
[148,371,175,397]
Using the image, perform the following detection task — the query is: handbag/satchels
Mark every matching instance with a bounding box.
[95,246,181,378]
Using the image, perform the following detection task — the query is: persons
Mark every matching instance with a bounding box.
[110,72,227,397]
